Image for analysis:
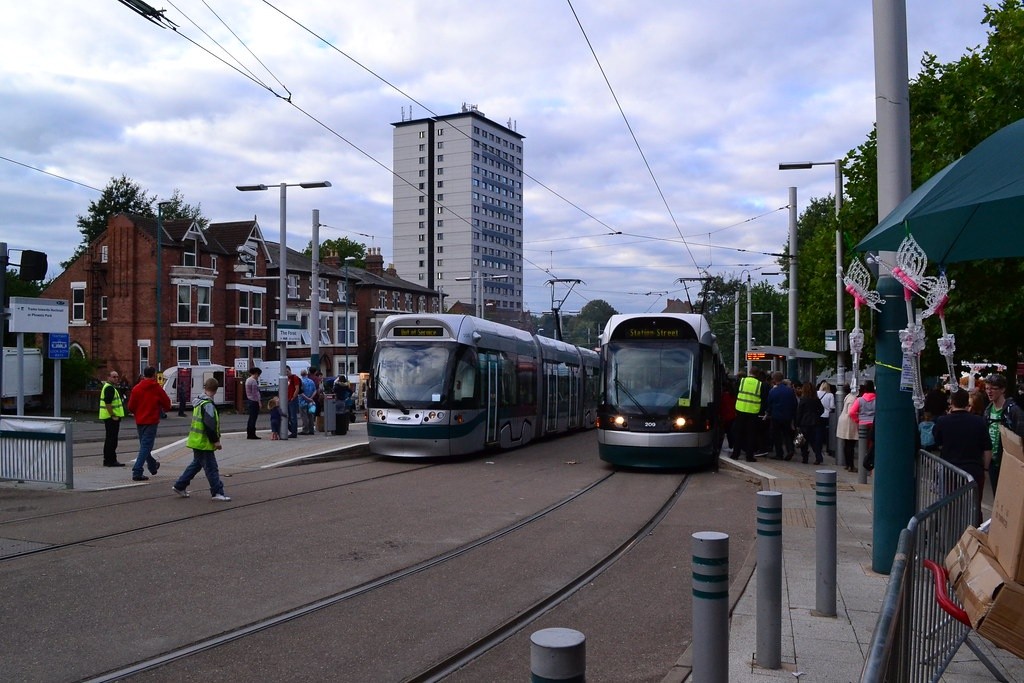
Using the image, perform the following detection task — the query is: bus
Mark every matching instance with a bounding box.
[366,312,595,460]
[595,312,729,474]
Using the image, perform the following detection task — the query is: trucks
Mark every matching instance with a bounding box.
[258,360,311,393]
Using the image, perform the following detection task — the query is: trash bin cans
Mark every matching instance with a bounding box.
[324,394,336,432]
[828,408,837,451]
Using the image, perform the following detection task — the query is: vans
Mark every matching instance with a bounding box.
[158,365,236,409]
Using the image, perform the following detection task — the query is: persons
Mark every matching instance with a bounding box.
[99,370,127,467]
[172,377,231,501]
[128,367,171,481]
[717,367,1024,552]
[643,372,669,391]
[91,366,370,440]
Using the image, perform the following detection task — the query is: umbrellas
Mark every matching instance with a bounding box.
[847,118,1024,269]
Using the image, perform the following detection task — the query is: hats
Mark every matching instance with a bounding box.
[338,376,348,382]
[983,375,1006,386]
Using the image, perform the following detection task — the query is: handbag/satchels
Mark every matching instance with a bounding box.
[793,433,807,449]
[308,401,316,414]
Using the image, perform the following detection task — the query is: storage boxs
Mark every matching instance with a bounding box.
[945,425,1024,660]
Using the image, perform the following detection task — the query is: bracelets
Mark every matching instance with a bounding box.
[111,415,115,417]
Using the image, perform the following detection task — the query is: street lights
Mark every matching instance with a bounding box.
[541,310,580,337]
[778,158,848,466]
[454,274,509,319]
[235,180,332,440]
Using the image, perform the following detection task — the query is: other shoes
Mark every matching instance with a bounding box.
[288,434,297,439]
[813,458,824,465]
[298,431,314,435]
[103,461,126,467]
[247,433,262,439]
[785,452,795,461]
[172,486,190,497]
[754,452,767,458]
[212,494,232,501]
[731,455,738,460]
[152,461,160,475]
[745,456,757,462]
[133,476,150,481]
[845,465,858,472]
[801,457,809,463]
[772,453,782,460]
[271,433,280,440]
